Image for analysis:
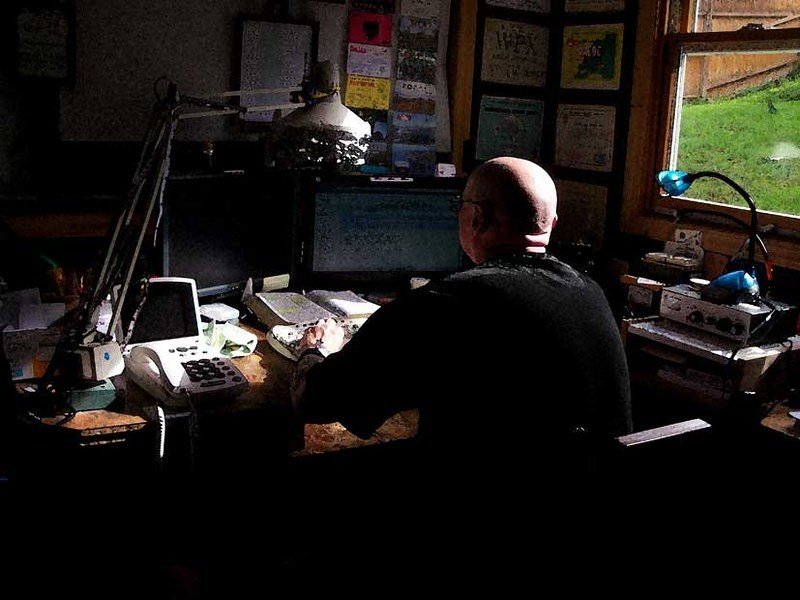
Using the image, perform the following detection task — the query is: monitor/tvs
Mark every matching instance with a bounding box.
[159,171,295,301]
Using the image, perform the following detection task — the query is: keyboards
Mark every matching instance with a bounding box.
[267,319,363,360]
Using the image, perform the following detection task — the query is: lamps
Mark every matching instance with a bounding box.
[49,56,376,385]
[649,165,775,307]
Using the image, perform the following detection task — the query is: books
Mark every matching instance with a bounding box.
[243,287,382,329]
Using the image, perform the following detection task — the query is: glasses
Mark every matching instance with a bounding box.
[452,195,480,210]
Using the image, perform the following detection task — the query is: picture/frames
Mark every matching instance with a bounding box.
[466,0,639,193]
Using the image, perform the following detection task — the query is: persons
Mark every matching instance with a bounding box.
[292,158,643,459]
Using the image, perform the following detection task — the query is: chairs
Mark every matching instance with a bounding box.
[508,416,712,600]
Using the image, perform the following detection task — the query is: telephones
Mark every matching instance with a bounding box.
[123,336,248,409]
